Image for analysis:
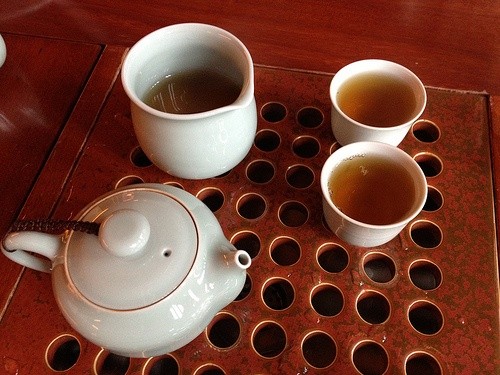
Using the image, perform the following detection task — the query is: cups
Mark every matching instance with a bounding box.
[320,141,427,247]
[330,59,427,148]
[121,23,256,180]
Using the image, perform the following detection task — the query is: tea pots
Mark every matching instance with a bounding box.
[0,183,251,358]
